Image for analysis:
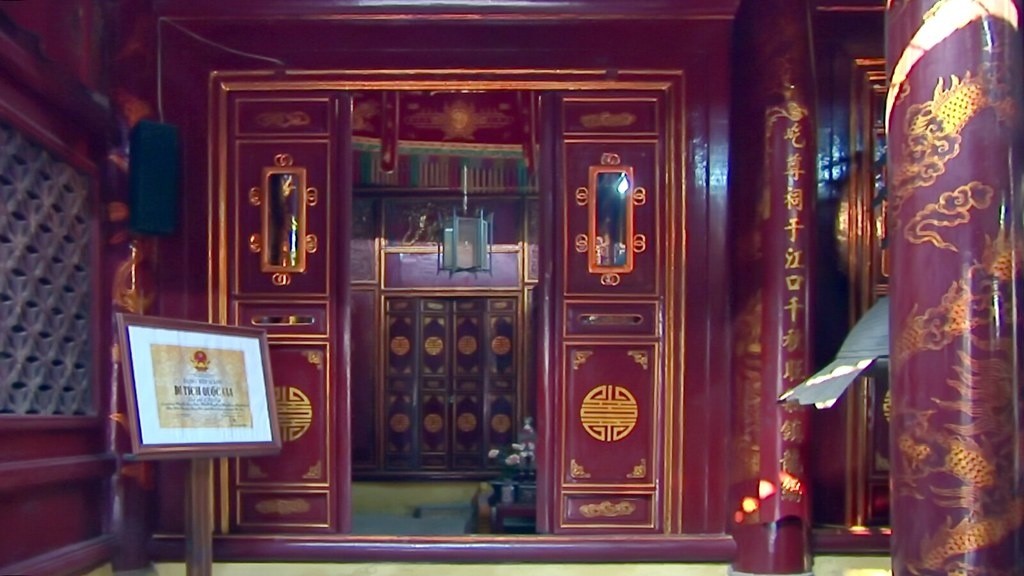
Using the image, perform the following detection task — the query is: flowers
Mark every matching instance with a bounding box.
[488,421,535,483]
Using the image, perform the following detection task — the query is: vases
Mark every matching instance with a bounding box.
[516,480,536,503]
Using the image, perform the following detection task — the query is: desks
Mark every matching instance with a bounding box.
[497,503,535,530]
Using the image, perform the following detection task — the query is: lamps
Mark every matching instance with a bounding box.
[433,164,496,282]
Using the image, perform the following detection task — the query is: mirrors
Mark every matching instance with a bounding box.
[261,165,307,274]
[589,164,635,273]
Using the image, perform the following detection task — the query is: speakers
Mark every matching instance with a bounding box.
[130,119,181,235]
